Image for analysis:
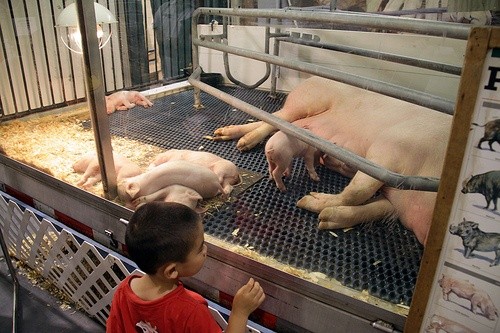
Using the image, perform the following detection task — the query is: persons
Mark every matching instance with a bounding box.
[104,201,266,333]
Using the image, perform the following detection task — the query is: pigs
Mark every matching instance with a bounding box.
[212,72,453,248]
[148,148,242,201]
[449,217,500,267]
[425,315,474,333]
[132,184,209,214]
[104,90,154,114]
[439,274,499,320]
[472,119,500,151]
[116,160,229,208]
[70,153,142,199]
[264,126,325,191]
[461,170,500,211]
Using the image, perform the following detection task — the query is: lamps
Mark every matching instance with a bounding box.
[57,1,118,54]
